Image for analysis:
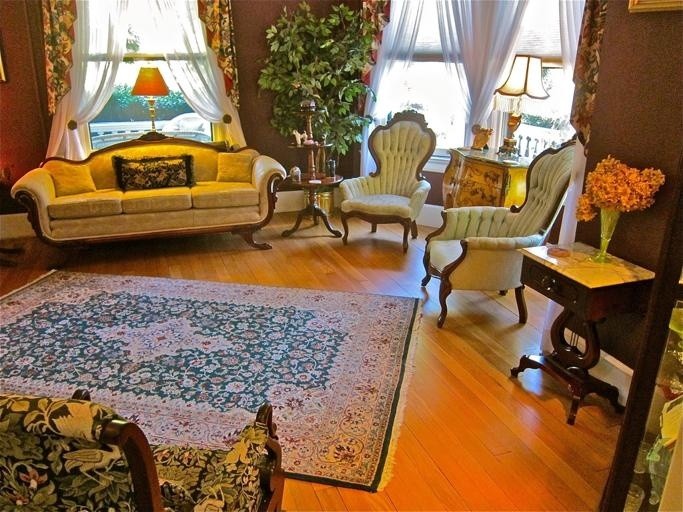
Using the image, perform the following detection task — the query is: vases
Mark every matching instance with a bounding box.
[589,209,619,264]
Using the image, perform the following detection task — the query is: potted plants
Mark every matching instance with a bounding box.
[257,2,381,218]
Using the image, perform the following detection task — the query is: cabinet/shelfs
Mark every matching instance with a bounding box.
[441,147,532,209]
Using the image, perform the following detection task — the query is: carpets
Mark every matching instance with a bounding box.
[0,270,423,493]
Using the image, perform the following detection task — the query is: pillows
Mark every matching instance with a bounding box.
[217,151,252,183]
[50,164,97,198]
[112,154,197,192]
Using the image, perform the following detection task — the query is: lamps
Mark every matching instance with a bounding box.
[493,55,550,156]
[132,66,169,132]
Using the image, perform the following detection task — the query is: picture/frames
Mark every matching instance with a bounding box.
[628,0,683,14]
[0,50,8,83]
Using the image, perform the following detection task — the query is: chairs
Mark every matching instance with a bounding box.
[339,109,436,254]
[0,389,287,512]
[421,134,578,329]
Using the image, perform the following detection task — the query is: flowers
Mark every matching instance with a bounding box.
[576,154,665,221]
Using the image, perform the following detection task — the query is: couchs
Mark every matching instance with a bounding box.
[11,132,287,270]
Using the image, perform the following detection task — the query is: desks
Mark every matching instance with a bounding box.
[510,241,656,425]
[282,175,343,238]
